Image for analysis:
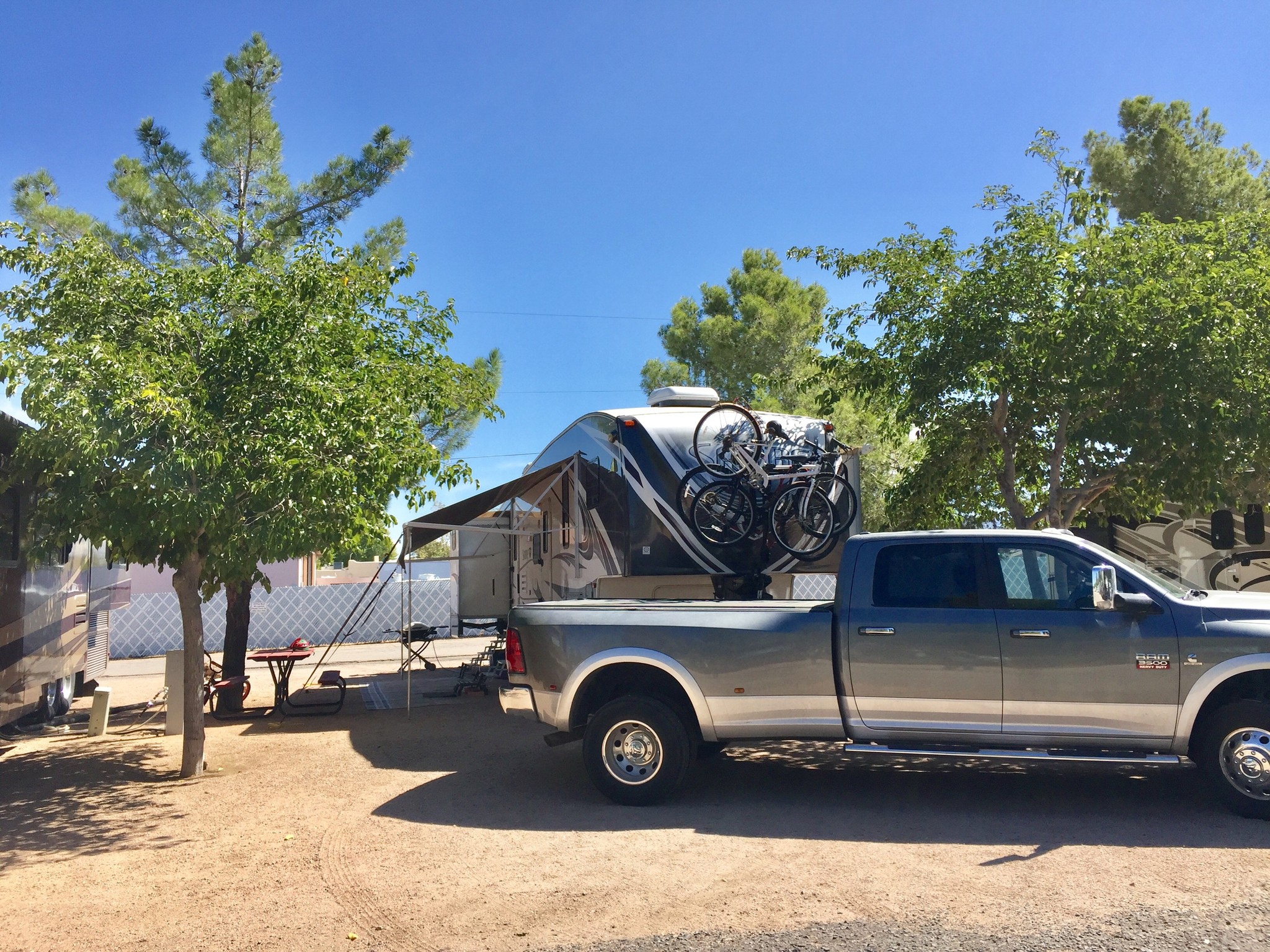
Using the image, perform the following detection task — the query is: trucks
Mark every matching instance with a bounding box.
[396,385,861,633]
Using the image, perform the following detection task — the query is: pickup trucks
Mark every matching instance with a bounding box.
[502,523,1270,824]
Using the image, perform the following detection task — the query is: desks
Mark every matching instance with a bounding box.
[247,648,318,718]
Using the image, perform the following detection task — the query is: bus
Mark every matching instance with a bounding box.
[0,408,131,738]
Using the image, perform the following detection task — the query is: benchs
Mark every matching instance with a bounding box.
[209,676,250,721]
[318,670,346,715]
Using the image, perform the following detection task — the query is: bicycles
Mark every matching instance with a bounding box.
[203,649,251,711]
[675,395,858,563]
